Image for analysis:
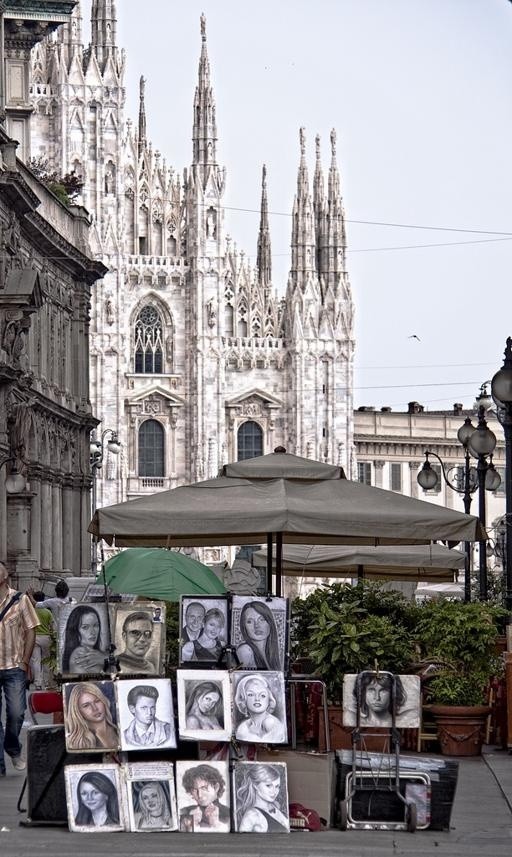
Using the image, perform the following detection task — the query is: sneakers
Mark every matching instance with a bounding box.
[12,755,26,771]
[36,686,42,690]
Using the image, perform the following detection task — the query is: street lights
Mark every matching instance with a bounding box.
[0,455,26,497]
[91,427,124,575]
[417,338,511,613]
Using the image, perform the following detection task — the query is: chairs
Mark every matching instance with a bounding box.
[16,690,63,813]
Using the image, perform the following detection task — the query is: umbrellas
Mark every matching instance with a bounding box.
[93,546,228,603]
[250,540,468,609]
[87,443,488,598]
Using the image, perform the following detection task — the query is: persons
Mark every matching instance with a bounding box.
[180,815,193,832]
[238,762,289,832]
[67,682,119,749]
[26,590,57,691]
[74,771,120,827]
[63,605,109,675]
[343,672,421,729]
[26,580,71,630]
[124,684,171,749]
[184,681,226,731]
[1,560,42,778]
[153,608,161,621]
[115,611,157,675]
[235,600,281,670]
[179,764,231,830]
[183,602,227,662]
[234,674,286,744]
[134,780,173,830]
